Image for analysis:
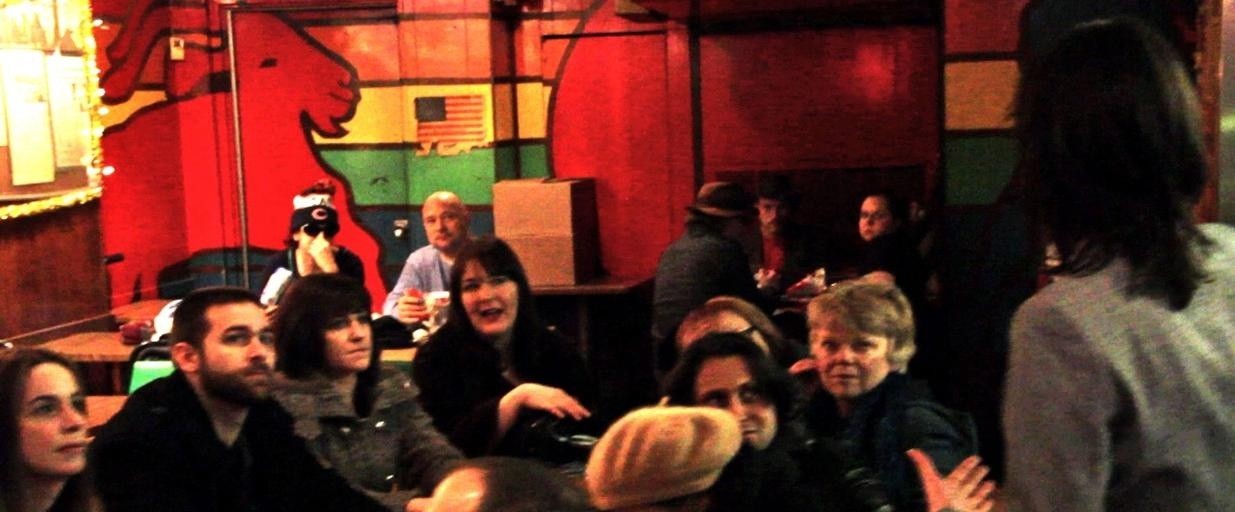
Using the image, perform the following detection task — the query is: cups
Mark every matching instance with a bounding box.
[424,291,451,335]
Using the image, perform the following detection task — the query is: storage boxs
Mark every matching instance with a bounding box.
[491,177,596,286]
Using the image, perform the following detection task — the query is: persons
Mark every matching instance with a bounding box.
[1,343,96,511]
[904,3,1235,511]
[89,172,1009,510]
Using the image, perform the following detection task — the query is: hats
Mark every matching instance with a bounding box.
[687,183,761,220]
[586,395,743,510]
